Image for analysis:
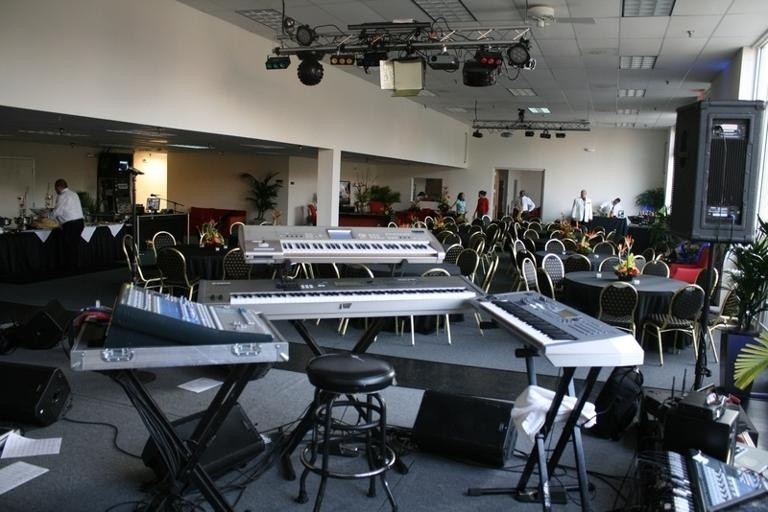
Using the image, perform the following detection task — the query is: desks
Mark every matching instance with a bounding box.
[0,219,131,287]
[66,308,276,512]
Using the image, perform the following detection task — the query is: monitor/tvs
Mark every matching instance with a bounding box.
[147,198,160,210]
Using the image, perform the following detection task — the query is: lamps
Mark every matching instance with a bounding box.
[262,0,538,100]
[469,105,568,140]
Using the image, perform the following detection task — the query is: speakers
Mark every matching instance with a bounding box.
[0,361,72,428]
[141,400,266,489]
[665,410,738,465]
[671,100,766,244]
[16,300,68,349]
[409,390,518,466]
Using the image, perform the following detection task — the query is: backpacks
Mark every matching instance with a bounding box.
[585,364,647,441]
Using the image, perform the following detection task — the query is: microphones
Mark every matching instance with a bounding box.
[150,193,157,198]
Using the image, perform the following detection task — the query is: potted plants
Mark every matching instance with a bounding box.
[714,212,767,397]
[237,168,286,226]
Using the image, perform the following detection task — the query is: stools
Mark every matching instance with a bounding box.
[293,349,404,511]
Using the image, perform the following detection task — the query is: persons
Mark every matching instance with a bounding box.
[472,190,489,221]
[511,190,536,218]
[570,190,593,228]
[416,192,425,203]
[598,198,621,217]
[448,192,468,218]
[38,179,86,240]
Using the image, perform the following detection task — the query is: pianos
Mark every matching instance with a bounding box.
[471,291,646,366]
[237,227,445,268]
[200,276,485,318]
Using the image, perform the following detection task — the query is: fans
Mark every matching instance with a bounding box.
[505,0,597,28]
